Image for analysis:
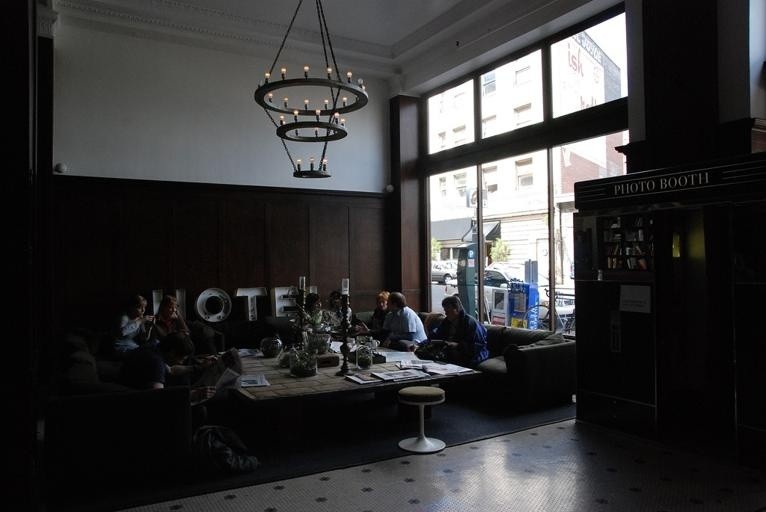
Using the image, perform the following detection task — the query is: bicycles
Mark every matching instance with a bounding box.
[522,288,575,335]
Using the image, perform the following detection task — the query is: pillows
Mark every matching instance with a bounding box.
[517,331,566,349]
[484,325,507,356]
[422,313,444,339]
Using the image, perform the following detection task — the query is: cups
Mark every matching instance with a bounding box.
[356,335,373,371]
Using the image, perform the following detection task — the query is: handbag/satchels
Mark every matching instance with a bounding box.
[193,425,253,474]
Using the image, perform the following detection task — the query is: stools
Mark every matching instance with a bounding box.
[398,386,445,454]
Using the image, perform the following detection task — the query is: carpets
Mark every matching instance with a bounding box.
[48,396,576,512]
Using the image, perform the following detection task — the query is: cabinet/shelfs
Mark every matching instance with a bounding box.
[596,215,650,272]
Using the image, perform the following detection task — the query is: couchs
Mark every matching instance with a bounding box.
[225,313,358,348]
[369,311,576,416]
[52,317,226,487]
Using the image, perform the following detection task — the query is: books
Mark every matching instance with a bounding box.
[343,359,475,385]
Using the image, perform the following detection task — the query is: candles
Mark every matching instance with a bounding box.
[299,276,305,290]
[342,278,349,294]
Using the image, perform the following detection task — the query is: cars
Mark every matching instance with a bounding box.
[484,264,576,320]
[431,259,458,284]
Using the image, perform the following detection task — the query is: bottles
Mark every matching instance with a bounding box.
[597,269,603,280]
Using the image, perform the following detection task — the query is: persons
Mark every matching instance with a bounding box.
[353,290,429,353]
[105,293,155,353]
[411,295,491,403]
[289,292,335,344]
[327,291,361,341]
[116,332,219,430]
[354,289,391,346]
[151,294,192,354]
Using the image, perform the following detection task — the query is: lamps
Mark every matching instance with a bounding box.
[253,1,369,179]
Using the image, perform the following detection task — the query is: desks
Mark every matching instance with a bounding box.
[219,339,482,401]
